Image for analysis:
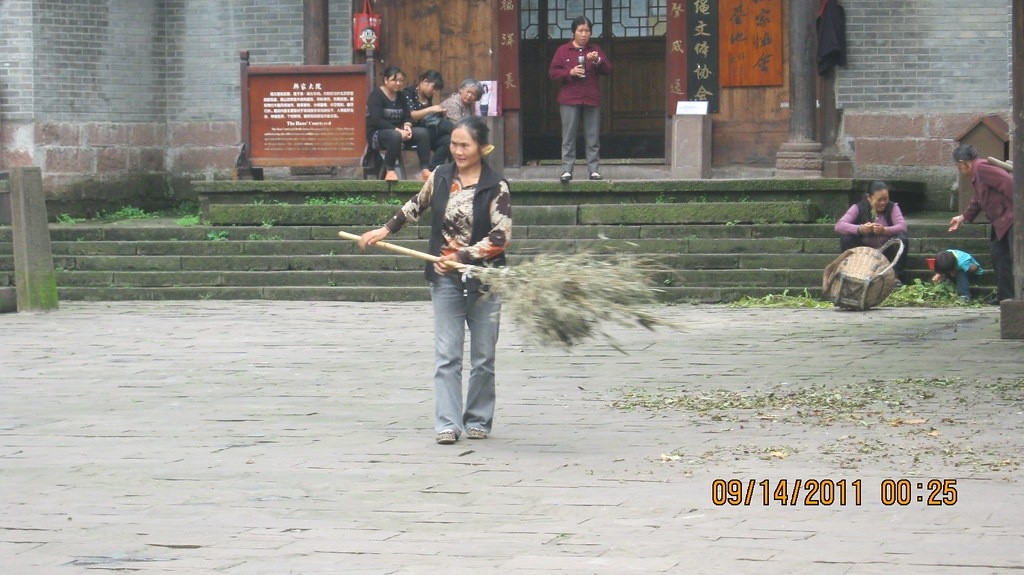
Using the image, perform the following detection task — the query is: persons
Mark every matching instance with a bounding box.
[401,69,495,172]
[947,144,1016,306]
[365,65,432,181]
[357,117,511,445]
[549,15,611,182]
[834,181,909,286]
[930,249,984,302]
[439,78,485,126]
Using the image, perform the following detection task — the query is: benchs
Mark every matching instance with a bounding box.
[380,143,418,179]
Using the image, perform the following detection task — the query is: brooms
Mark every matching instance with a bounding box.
[337,229,692,358]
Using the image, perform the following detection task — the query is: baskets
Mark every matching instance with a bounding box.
[822,236,905,311]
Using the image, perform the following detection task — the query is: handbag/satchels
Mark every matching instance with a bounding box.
[353,0,381,51]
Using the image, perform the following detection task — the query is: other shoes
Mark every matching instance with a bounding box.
[467,428,486,440]
[960,295,971,304]
[385,171,398,181]
[590,172,602,179]
[436,432,456,443]
[560,172,572,181]
[420,170,431,179]
